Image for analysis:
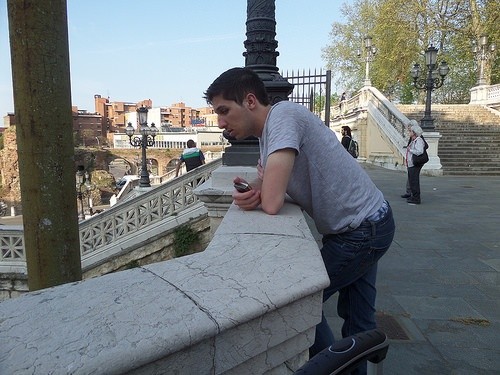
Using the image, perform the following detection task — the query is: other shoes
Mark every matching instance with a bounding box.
[401,194,411,198]
[407,197,420,204]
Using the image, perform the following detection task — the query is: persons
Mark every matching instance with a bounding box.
[341,92,347,100]
[341,126,353,152]
[203,67,394,375]
[175,140,205,201]
[406,125,425,203]
[400,120,429,198]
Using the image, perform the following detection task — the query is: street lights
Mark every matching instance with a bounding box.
[84,171,93,215]
[356,34,377,86]
[75,170,85,221]
[125,103,161,188]
[472,32,495,86]
[408,43,450,132]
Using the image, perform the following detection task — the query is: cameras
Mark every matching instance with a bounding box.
[235,182,252,193]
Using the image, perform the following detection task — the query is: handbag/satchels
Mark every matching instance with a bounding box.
[413,152,429,169]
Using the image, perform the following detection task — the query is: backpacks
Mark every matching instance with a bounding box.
[343,136,359,158]
